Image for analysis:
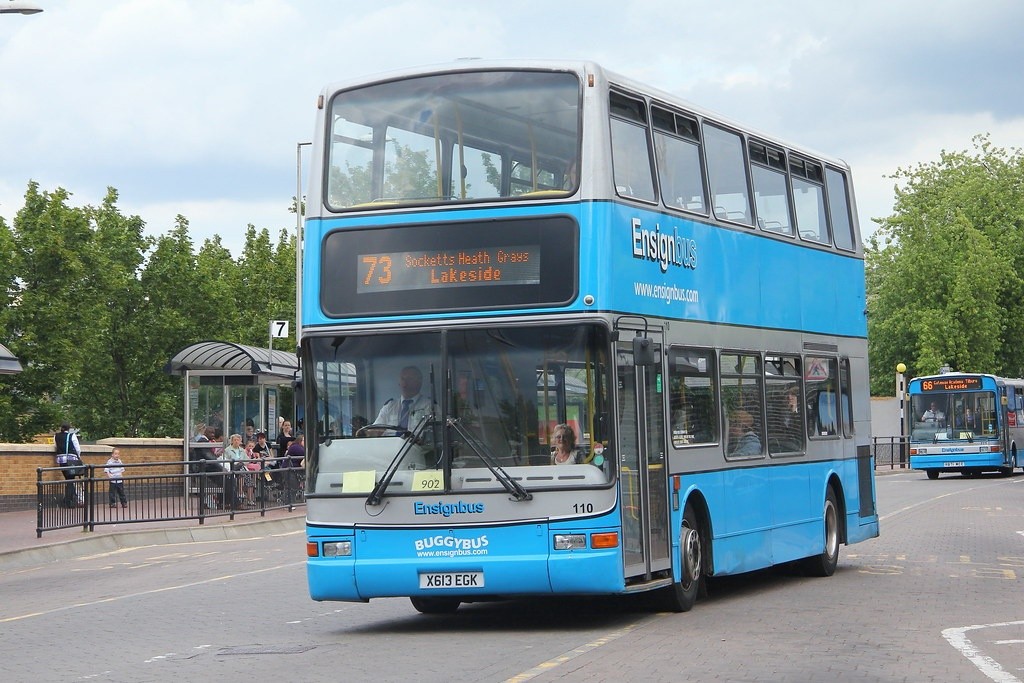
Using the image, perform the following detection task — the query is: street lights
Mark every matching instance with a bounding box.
[897,362,907,468]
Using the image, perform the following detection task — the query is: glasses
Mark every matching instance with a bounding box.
[555,436,570,439]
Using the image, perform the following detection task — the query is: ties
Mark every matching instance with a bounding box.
[933,411,936,421]
[399,400,409,437]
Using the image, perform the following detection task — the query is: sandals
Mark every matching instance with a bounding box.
[238,493,247,498]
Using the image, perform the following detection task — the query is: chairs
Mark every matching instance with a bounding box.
[680,201,818,242]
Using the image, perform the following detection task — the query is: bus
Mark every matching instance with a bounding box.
[298,61,880,613]
[908,373,1024,480]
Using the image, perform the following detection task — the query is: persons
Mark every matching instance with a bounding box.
[920,401,946,423]
[53,422,81,508]
[565,159,578,192]
[364,366,443,437]
[550,423,586,465]
[963,408,973,421]
[667,376,713,442]
[189,417,306,510]
[772,386,814,442]
[728,409,763,457]
[330,423,337,436]
[105,448,127,508]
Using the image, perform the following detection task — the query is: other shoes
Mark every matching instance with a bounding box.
[62,501,68,509]
[217,503,231,510]
[122,503,128,508]
[109,503,117,508]
[247,501,257,505]
[234,504,250,510]
[69,501,76,508]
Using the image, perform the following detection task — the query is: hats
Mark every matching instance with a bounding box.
[728,409,755,430]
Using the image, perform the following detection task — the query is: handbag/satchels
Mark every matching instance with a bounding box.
[283,467,301,490]
[65,460,87,476]
[56,454,79,464]
